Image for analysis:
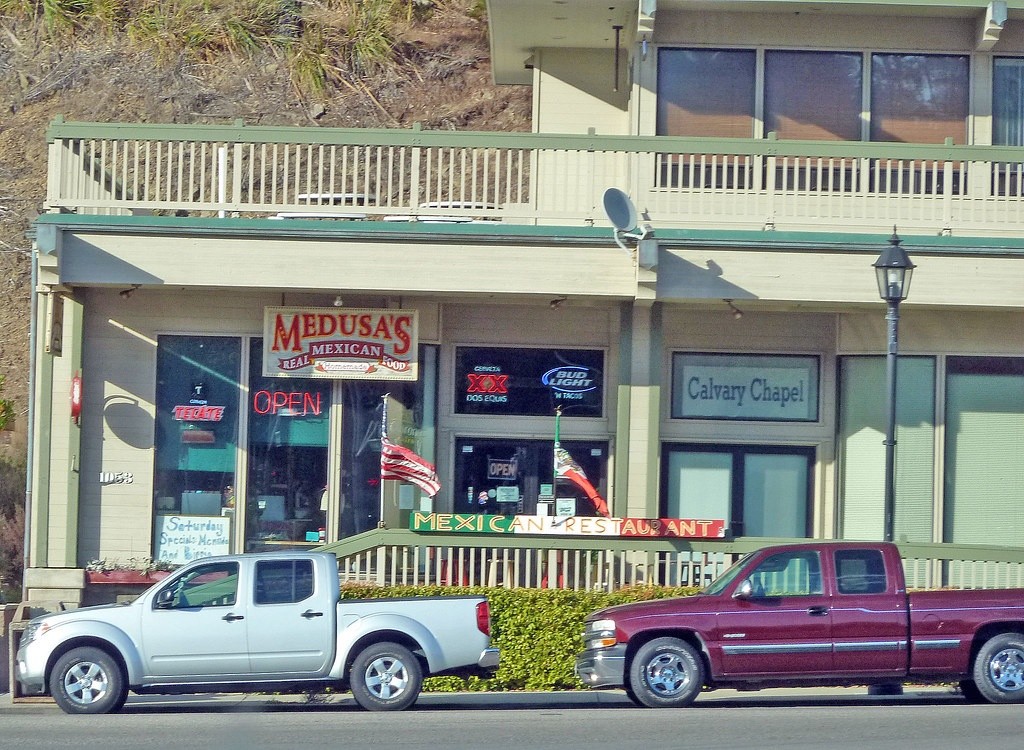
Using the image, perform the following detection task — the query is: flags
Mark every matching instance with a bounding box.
[553,447,609,518]
[380,432,442,498]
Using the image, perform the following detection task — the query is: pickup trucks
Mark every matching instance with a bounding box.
[13,550,500,711]
[576,541,1024,709]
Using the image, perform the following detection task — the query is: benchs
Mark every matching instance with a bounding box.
[383,216,473,223]
[278,213,367,222]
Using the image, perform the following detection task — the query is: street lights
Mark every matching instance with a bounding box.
[867,223,918,696]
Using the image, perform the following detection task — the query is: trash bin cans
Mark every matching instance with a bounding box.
[9,600,62,703]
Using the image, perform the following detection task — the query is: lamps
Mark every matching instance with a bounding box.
[722,298,745,321]
[120,284,142,301]
[549,296,567,312]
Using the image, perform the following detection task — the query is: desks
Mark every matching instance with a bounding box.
[420,202,504,208]
[298,193,376,208]
